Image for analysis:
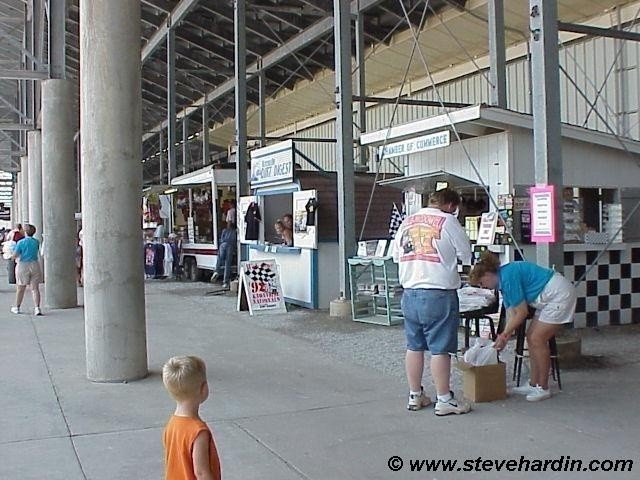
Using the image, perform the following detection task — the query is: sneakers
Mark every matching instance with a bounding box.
[222,282,228,288]
[512,378,552,401]
[211,271,219,283]
[434,391,471,416]
[11,306,21,314]
[34,307,42,316]
[406,386,432,411]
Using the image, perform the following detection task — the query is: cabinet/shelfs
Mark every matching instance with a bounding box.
[348,255,405,327]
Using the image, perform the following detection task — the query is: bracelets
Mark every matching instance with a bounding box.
[497,333,510,340]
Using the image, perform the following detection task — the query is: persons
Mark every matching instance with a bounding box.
[162,355,221,480]
[274,220,292,246]
[284,215,293,228]
[393,189,472,416]
[11,225,42,316]
[469,250,578,401]
[7,224,25,242]
[211,223,237,288]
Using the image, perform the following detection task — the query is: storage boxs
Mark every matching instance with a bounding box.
[454,347,506,404]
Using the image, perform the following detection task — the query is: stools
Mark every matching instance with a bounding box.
[512,307,564,392]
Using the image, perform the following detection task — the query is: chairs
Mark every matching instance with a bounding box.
[455,284,499,348]
